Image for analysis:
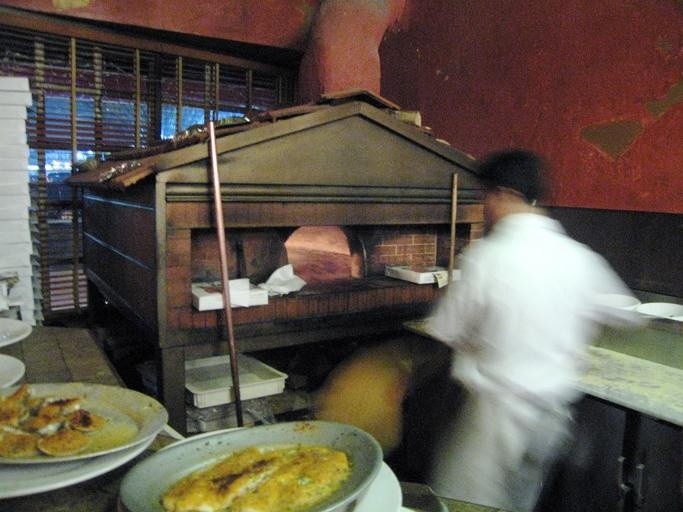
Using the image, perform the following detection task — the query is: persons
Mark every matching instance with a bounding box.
[388,149,652,511]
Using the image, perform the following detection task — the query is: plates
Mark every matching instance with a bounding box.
[0,353,26,390]
[594,293,641,311]
[636,302,683,323]
[0,379,171,501]
[117,419,404,512]
[0,316,32,349]
